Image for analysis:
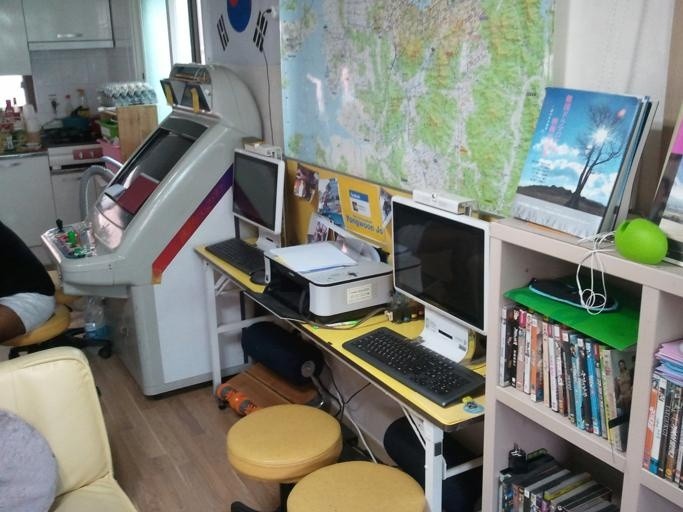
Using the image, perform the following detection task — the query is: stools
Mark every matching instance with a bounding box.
[225,403,343,512]
[283,460,427,512]
[7,284,108,360]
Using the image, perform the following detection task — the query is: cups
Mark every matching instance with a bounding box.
[26,120,41,145]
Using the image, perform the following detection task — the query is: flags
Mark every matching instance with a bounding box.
[209,1,271,65]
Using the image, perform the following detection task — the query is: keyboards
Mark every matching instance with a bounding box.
[206,237,264,275]
[342,326,485,408]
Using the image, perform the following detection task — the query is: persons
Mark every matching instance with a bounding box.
[0,219,55,339]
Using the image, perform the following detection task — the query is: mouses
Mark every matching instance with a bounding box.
[251,271,265,284]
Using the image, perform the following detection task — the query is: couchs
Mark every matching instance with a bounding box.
[0,341,140,512]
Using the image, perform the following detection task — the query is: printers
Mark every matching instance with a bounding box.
[239,240,393,327]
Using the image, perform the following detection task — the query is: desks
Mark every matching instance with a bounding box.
[193,234,484,511]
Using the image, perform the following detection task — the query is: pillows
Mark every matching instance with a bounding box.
[0,409,60,512]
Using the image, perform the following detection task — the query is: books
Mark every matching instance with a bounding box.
[658,102,683,267]
[513,86,658,241]
[499,303,638,453]
[499,450,620,512]
[641,372,683,489]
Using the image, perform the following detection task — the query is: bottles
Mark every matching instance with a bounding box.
[101,83,157,113]
[78,92,90,118]
[64,95,73,118]
[84,296,108,340]
[5,98,24,129]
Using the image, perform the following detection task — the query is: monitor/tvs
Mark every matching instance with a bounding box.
[231,144,286,252]
[104,126,198,217]
[390,186,490,364]
